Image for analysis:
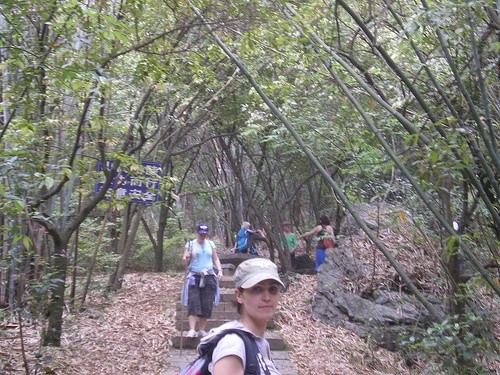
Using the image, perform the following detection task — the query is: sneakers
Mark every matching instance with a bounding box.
[186,329,198,338]
[197,329,207,338]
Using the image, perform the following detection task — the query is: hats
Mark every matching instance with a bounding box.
[197,225,209,236]
[234,258,285,289]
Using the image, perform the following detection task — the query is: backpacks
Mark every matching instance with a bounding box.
[178,328,260,375]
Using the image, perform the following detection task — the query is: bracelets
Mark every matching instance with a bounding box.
[218,270,223,271]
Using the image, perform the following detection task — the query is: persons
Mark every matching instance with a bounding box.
[182,222,224,338]
[233,215,338,275]
[177,258,286,375]
